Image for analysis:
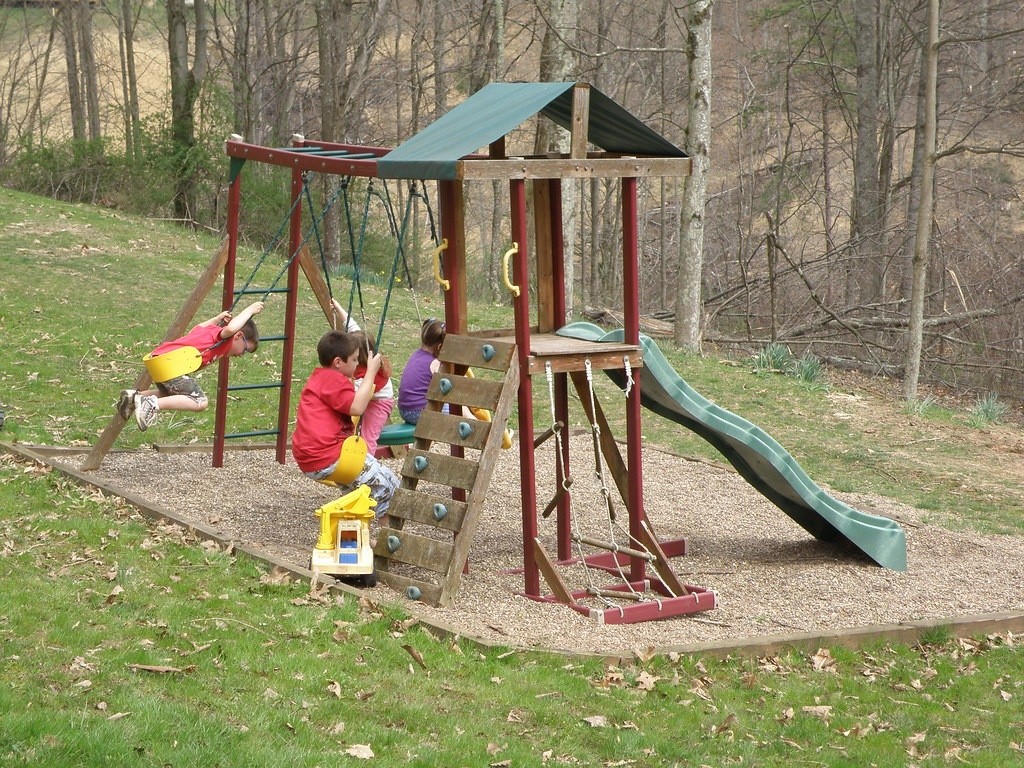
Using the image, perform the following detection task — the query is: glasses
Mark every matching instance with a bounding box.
[242,335,248,353]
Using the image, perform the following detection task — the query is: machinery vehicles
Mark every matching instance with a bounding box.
[309,485,378,588]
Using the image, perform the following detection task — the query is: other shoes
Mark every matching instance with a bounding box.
[506,428,514,438]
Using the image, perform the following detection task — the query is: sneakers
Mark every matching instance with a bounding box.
[135,395,160,432]
[117,390,139,421]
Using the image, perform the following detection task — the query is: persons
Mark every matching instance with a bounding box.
[117,300,264,432]
[397,318,450,425]
[290,330,399,529]
[330,296,394,459]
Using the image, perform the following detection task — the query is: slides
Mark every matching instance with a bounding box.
[558,318,909,572]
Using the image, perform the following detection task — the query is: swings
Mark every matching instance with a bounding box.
[144,175,349,383]
[301,173,447,489]
